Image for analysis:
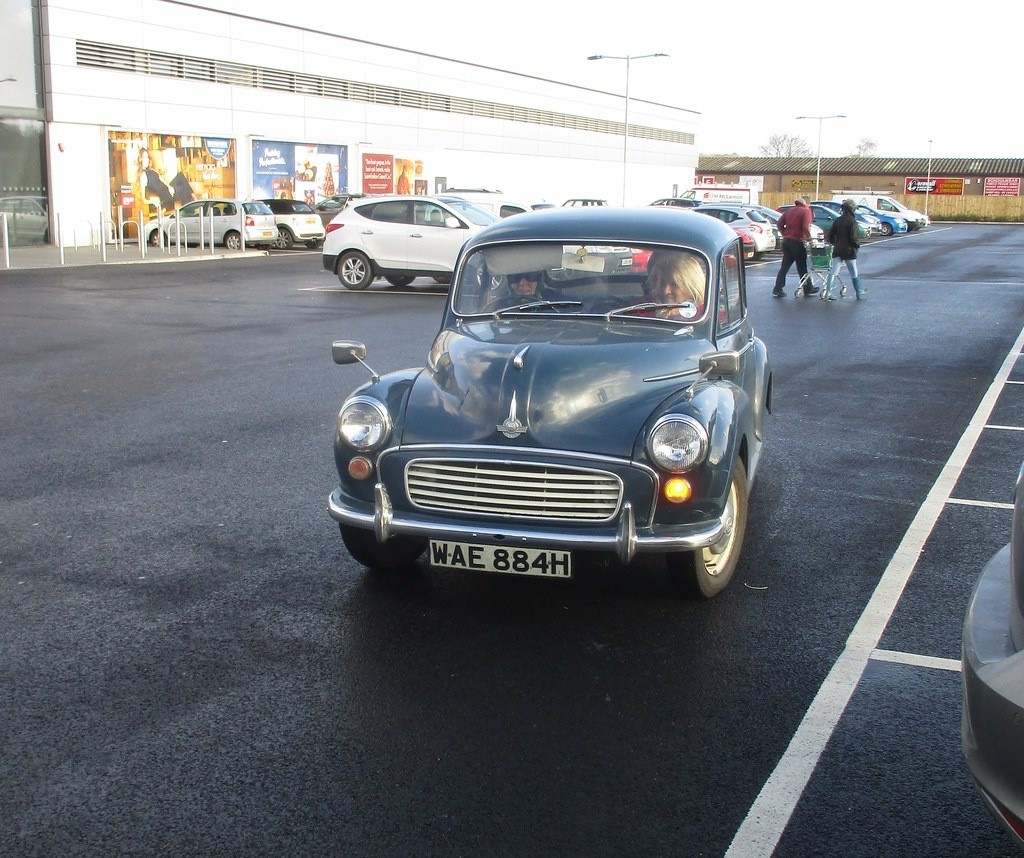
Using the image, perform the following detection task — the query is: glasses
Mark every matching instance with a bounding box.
[506,273,541,283]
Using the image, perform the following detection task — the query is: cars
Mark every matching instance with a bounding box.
[855,203,909,237]
[324,201,775,597]
[253,198,325,249]
[632,228,755,271]
[310,195,367,225]
[957,454,1024,850]
[561,198,614,209]
[1,193,47,244]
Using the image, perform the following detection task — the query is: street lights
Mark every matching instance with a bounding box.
[797,114,846,200]
[925,139,933,216]
[587,53,673,206]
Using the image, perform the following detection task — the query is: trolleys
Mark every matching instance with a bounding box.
[794,236,849,300]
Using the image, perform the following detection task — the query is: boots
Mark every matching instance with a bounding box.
[852,276,868,300]
[826,275,836,300]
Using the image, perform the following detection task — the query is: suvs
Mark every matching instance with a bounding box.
[641,199,705,210]
[445,189,632,279]
[683,204,776,260]
[323,195,504,290]
[776,203,869,242]
[811,200,884,237]
[142,199,279,250]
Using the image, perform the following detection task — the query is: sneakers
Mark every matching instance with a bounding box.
[806,287,819,295]
[773,292,787,298]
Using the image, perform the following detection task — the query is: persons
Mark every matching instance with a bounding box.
[137,148,198,209]
[825,199,863,300]
[397,164,412,195]
[772,196,821,297]
[478,271,577,314]
[627,255,725,326]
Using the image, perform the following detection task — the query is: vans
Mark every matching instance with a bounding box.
[718,202,826,249]
[677,185,760,210]
[829,189,929,232]
